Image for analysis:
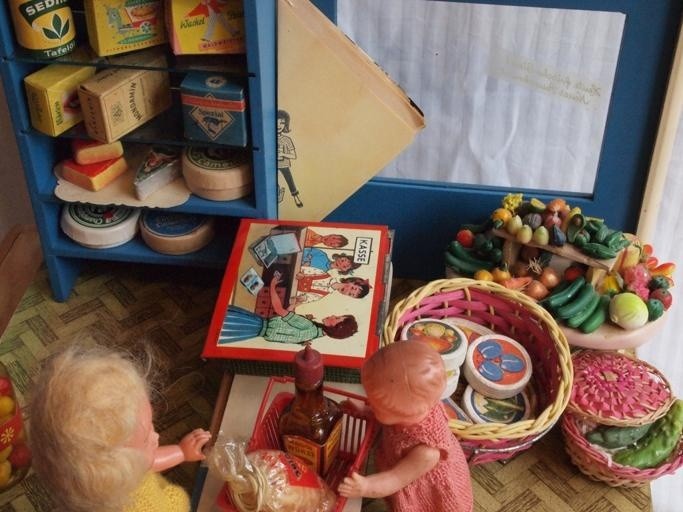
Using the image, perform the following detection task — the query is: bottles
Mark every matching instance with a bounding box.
[277,344,343,480]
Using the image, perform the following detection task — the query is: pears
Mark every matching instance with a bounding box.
[508,215,549,246]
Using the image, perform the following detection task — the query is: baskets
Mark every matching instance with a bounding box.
[215,374,378,511]
[562,347,683,489]
[378,277,575,468]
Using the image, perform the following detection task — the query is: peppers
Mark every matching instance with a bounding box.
[492,262,511,282]
[473,269,493,281]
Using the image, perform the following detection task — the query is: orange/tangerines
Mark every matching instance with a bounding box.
[646,298,664,321]
[493,208,512,225]
[650,275,669,289]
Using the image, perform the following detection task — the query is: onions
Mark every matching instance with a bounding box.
[608,292,649,330]
[513,245,581,299]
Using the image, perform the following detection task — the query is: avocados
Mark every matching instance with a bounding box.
[567,213,631,258]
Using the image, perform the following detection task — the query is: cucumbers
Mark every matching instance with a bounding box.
[544,276,610,333]
[443,218,504,274]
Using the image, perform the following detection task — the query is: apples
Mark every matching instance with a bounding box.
[651,287,672,310]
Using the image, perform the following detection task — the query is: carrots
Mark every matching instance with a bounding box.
[502,277,533,290]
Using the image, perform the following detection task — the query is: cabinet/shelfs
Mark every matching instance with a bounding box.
[1,1,280,303]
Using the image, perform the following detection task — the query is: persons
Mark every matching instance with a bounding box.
[301,246,361,273]
[28,322,211,511]
[218,271,357,346]
[304,228,348,248]
[294,264,372,304]
[339,341,474,512]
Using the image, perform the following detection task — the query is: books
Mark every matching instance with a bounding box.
[200,218,388,368]
[277,1,426,224]
